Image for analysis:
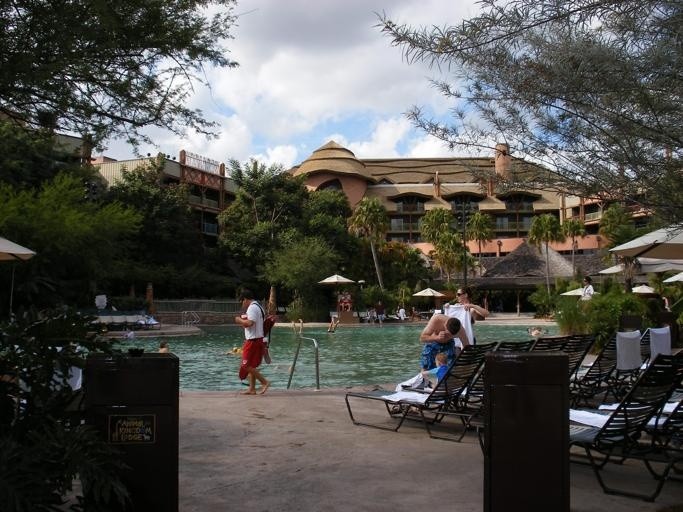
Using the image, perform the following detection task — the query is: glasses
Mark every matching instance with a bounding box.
[584,281,588,282]
[458,293,465,296]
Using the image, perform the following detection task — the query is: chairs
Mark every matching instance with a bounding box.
[92,312,163,332]
[568,349,682,503]
[329,308,442,322]
[344,329,672,443]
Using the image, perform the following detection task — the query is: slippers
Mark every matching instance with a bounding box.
[391,405,418,414]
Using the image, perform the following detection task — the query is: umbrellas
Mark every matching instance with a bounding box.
[607,222,683,260]
[661,271,683,283]
[410,287,446,311]
[559,287,600,297]
[316,274,356,294]
[0,236,37,263]
[597,257,683,275]
[622,283,655,294]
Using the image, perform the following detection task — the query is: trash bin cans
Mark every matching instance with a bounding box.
[84,350,177,511]
[485,352,570,511]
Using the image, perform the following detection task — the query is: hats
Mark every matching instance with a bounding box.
[584,276,592,281]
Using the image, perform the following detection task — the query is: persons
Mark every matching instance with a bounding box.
[158,341,170,354]
[580,276,593,303]
[136,310,151,323]
[327,320,340,333]
[233,290,271,395]
[525,325,551,337]
[417,313,472,371]
[443,286,489,346]
[418,353,452,394]
[123,327,134,340]
[339,291,352,312]
[365,302,425,326]
